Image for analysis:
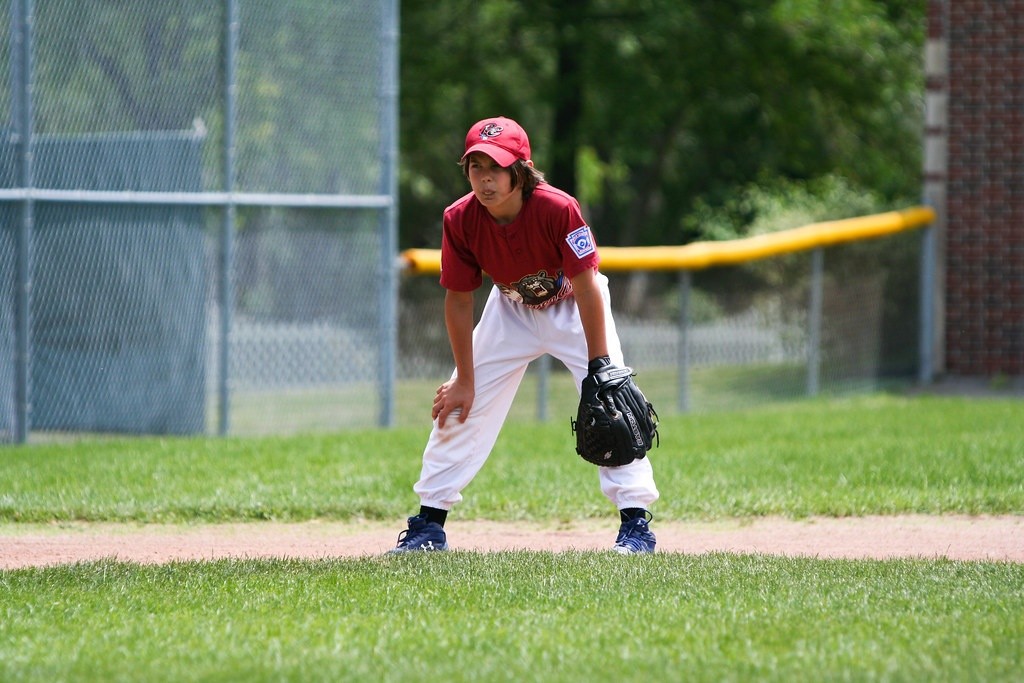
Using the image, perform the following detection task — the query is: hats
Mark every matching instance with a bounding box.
[461,116,530,167]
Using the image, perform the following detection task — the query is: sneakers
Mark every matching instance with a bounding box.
[613,509,657,555]
[395,514,449,553]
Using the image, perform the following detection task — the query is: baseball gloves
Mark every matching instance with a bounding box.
[569,354,662,469]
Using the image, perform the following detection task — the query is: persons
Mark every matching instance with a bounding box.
[387,116,659,555]
